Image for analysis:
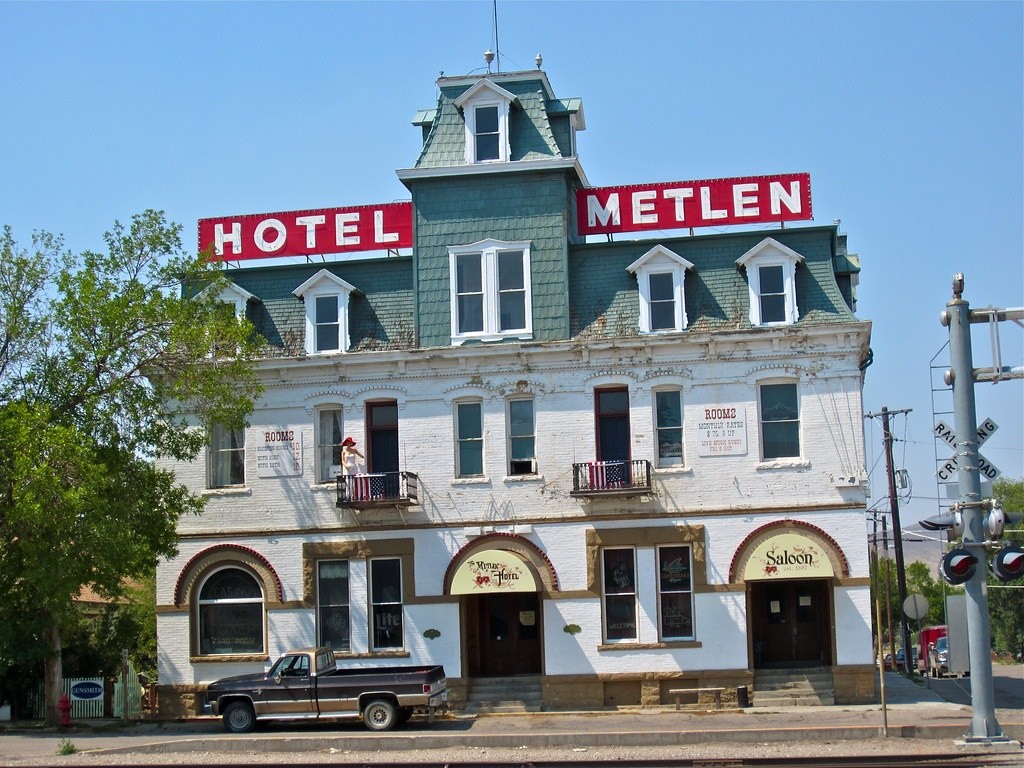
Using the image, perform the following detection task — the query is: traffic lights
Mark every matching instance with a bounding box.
[918,509,959,543]
[941,549,979,586]
[992,544,1024,581]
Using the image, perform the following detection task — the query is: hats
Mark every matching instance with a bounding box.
[342,437,356,447]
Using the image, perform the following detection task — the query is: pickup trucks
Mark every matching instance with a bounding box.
[204,647,450,732]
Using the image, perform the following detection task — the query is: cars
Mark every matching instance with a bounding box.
[884,653,898,671]
[927,637,970,678]
[896,648,919,670]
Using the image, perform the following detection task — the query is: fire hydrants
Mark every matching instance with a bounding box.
[57,695,73,726]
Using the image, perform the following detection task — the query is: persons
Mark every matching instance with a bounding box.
[341,436,364,502]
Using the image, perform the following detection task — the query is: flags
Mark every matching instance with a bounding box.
[353,472,385,500]
[588,460,622,490]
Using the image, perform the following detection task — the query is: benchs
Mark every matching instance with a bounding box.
[669,687,725,710]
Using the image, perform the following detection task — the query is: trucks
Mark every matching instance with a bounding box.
[917,625,948,677]
